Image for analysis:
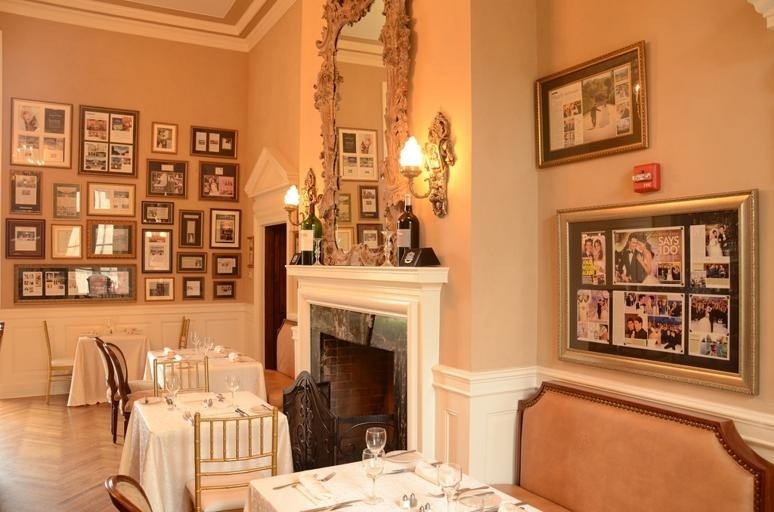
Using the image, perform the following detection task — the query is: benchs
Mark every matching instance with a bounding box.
[487,380,774,512]
[260,316,297,416]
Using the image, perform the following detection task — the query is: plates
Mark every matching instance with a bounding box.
[154,351,182,361]
[139,397,162,404]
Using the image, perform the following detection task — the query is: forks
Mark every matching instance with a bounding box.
[272,471,336,491]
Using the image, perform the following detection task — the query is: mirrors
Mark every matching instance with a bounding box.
[313,1,416,268]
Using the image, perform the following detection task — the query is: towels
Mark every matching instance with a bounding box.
[413,458,455,488]
[295,470,334,506]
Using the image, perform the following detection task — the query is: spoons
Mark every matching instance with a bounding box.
[181,411,196,426]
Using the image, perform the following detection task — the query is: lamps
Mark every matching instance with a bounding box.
[284,168,319,229]
[397,108,458,221]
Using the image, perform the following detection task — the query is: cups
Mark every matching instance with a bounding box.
[360,426,501,511]
[189,331,238,362]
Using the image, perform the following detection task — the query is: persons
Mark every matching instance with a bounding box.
[591,104,601,127]
[576,225,728,359]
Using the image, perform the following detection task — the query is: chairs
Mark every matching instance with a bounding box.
[94,315,272,443]
[185,406,284,512]
[104,474,155,512]
[42,320,78,404]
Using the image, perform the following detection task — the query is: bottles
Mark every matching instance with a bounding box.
[299,204,322,265]
[396,194,419,262]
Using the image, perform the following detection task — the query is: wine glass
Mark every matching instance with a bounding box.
[311,223,327,266]
[165,377,180,406]
[226,373,241,409]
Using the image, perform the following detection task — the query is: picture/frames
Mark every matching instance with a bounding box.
[76,103,141,179]
[211,280,237,300]
[150,120,180,155]
[85,218,138,261]
[180,275,207,301]
[196,158,241,204]
[531,36,651,170]
[144,157,190,200]
[6,218,47,260]
[175,250,209,274]
[143,276,177,302]
[187,124,239,160]
[211,252,243,280]
[140,198,175,226]
[140,228,175,274]
[178,208,205,248]
[553,189,762,401]
[13,263,140,306]
[207,206,242,250]
[8,96,74,170]
[335,123,384,253]
[86,180,136,218]
[51,223,84,260]
[6,171,45,217]
[50,180,83,221]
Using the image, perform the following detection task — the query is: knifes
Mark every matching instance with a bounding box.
[301,498,360,512]
[234,408,249,418]
